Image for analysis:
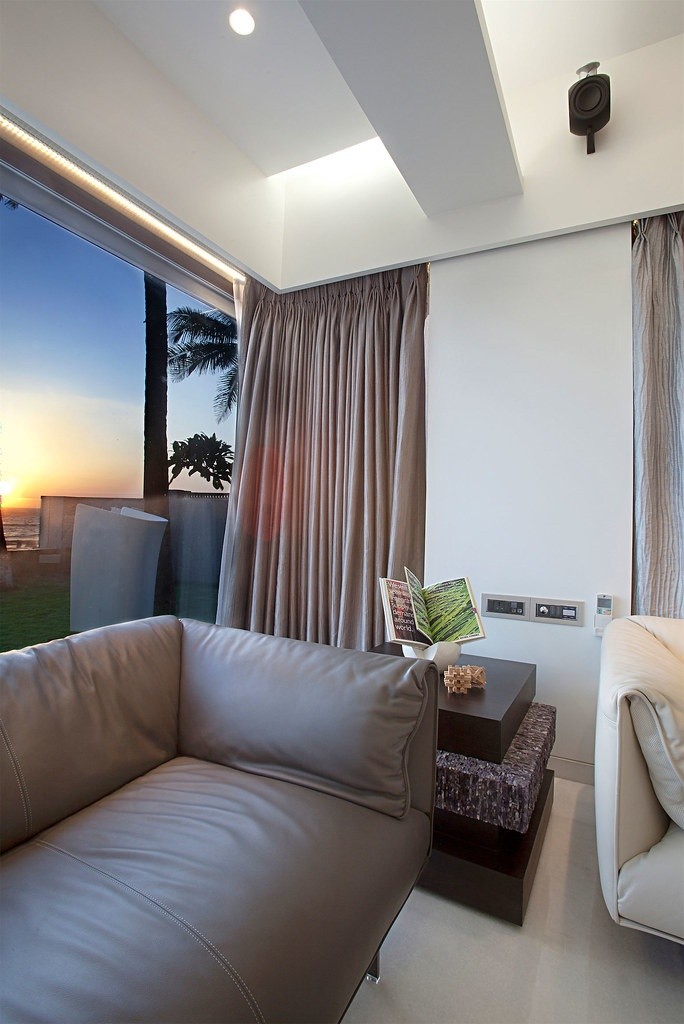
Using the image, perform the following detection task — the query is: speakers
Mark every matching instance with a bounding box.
[568,73,611,136]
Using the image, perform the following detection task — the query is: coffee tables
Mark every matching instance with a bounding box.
[367,641,556,928]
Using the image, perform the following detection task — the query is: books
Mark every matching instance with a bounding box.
[377,565,487,652]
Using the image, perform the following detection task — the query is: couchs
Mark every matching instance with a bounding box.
[591,615,684,946]
[0,614,439,1024]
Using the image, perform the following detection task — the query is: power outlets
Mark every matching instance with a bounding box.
[530,597,594,630]
[481,594,531,621]
